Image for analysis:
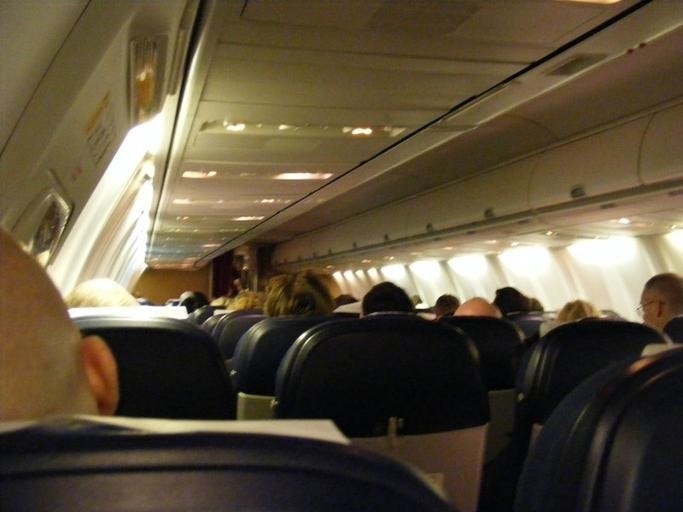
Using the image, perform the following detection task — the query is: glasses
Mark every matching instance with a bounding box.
[636,302,665,315]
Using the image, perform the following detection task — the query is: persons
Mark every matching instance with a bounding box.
[175,267,599,337]
[641,273,682,346]
[2,225,143,419]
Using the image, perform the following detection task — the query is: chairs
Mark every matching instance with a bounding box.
[1,291,683,507]
